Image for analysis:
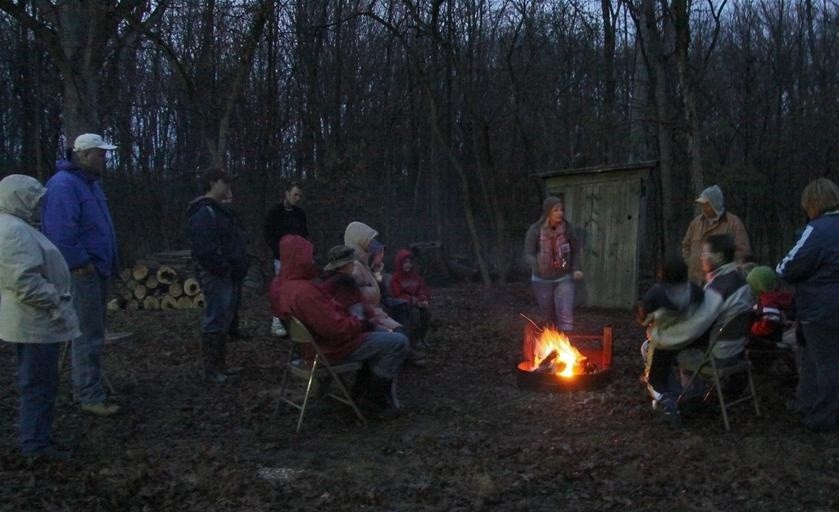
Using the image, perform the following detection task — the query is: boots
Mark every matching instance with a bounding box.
[203,332,228,383]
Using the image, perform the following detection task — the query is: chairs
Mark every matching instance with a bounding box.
[744,316,802,406]
[677,310,761,428]
[274,316,368,433]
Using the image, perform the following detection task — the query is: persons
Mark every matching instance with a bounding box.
[1,174,82,469]
[270,234,408,418]
[523,197,584,335]
[321,221,434,359]
[637,233,793,421]
[776,182,837,428]
[267,183,311,334]
[42,133,124,417]
[184,168,249,383]
[682,187,750,286]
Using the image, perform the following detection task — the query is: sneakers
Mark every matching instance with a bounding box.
[82,402,120,416]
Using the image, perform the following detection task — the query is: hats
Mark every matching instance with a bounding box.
[73,133,118,152]
[324,245,355,271]
